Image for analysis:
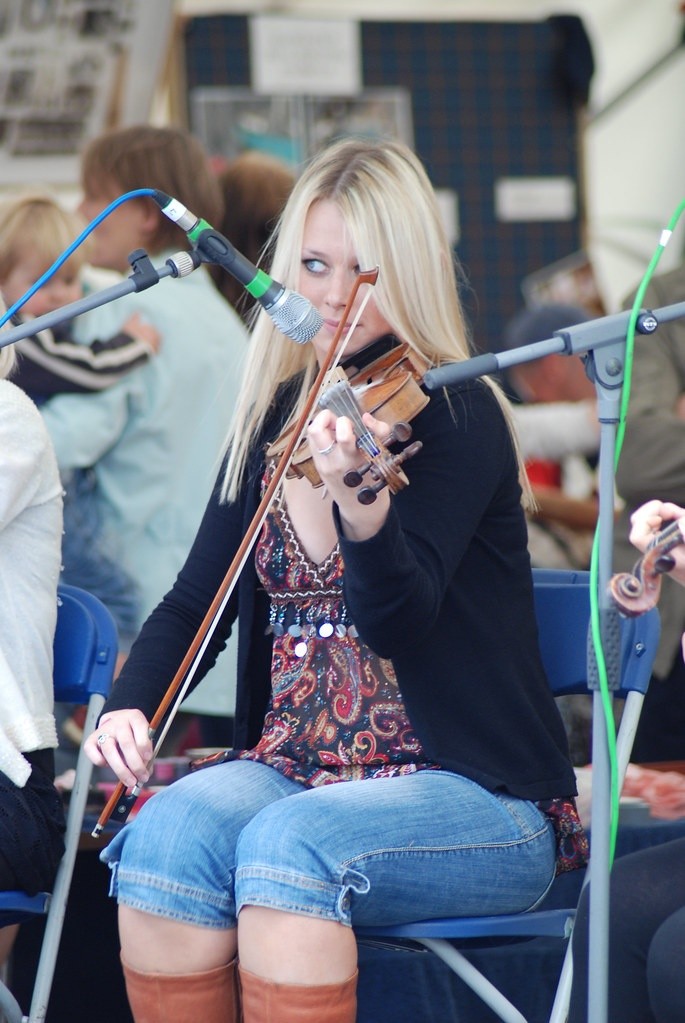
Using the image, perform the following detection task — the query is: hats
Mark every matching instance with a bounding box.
[494,304,587,349]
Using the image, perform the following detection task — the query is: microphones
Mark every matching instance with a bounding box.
[151,186,324,345]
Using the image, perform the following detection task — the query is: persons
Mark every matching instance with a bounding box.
[0,122,684,1022]
[77,137,588,1022]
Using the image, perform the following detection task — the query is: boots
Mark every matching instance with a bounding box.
[238,962,359,1023]
[120,954,238,1023]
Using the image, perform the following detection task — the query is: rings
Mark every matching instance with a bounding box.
[318,440,335,455]
[97,734,115,747]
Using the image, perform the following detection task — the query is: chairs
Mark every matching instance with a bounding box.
[354,566,658,1023]
[1,586,119,1022]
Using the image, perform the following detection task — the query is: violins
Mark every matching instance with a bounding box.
[603,520,683,618]
[268,343,431,504]
